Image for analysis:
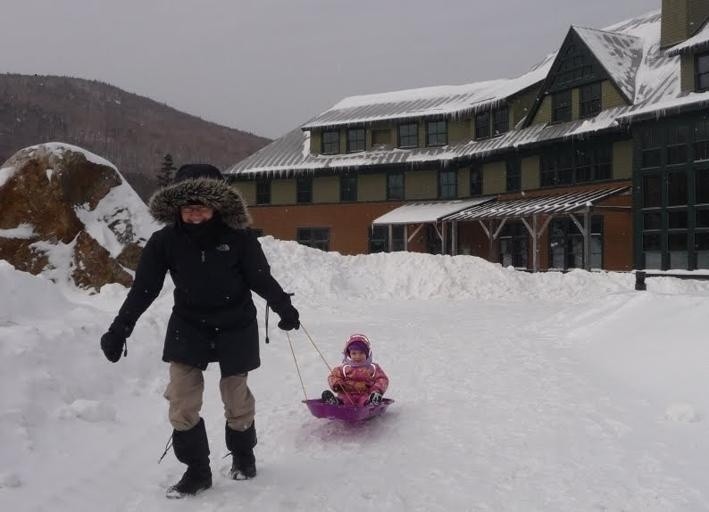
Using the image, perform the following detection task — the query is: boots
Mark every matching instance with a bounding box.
[165,416,212,499]
[225,419,257,480]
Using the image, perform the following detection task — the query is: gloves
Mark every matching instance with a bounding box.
[267,287,300,331]
[101,330,124,362]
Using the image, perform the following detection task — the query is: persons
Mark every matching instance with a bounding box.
[100,164,300,499]
[322,334,388,407]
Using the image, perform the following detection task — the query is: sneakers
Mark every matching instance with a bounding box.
[368,390,382,404]
[322,390,336,406]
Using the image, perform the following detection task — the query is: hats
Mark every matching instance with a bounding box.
[348,341,369,357]
